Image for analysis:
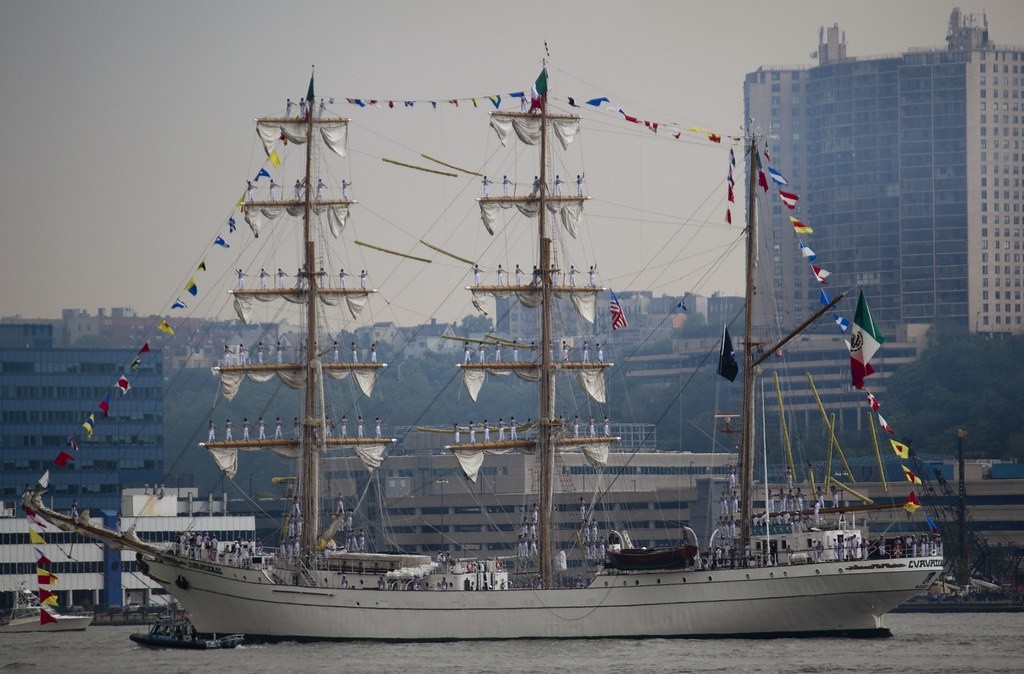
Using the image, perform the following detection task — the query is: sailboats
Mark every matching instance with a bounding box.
[19,40,945,639]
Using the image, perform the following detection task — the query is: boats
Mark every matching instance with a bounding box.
[605,544,700,566]
[129,619,246,648]
[0,582,94,633]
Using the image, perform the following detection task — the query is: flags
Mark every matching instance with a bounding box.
[531,67,549,111]
[304,72,314,100]
[183,610,193,619]
[623,111,922,517]
[39,134,288,490]
[609,287,628,331]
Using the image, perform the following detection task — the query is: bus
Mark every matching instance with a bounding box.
[497,550,568,584]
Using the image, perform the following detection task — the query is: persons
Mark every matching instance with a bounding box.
[682,524,687,543]
[183,625,197,640]
[125,592,132,612]
[176,496,452,591]
[207,96,384,442]
[452,92,609,444]
[701,463,941,569]
[71,497,79,518]
[24,483,33,498]
[516,497,605,589]
[115,513,121,532]
